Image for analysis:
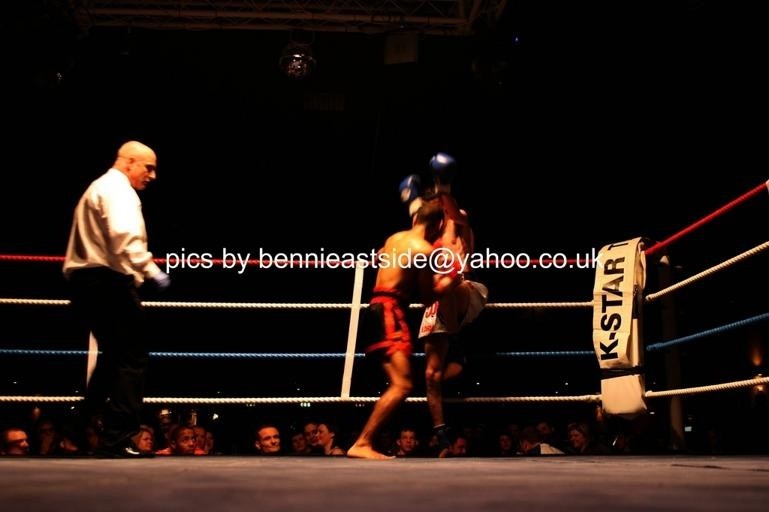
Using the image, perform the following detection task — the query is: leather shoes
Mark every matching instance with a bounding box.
[97,439,154,459]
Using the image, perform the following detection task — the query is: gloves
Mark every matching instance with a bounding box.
[399,175,421,204]
[429,152,455,184]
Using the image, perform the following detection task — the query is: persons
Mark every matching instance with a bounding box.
[399,151,487,443]
[345,205,462,459]
[62,139,170,459]
